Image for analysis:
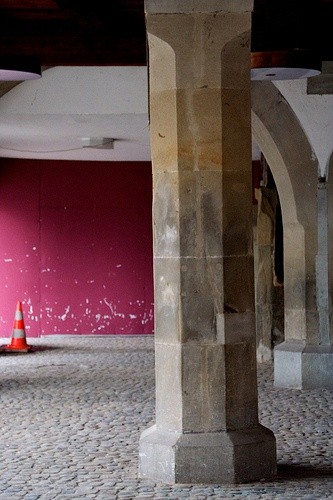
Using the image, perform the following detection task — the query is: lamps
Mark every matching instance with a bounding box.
[0,56,42,81]
[249,51,322,81]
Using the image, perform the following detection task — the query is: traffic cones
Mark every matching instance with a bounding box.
[1,301,32,350]
